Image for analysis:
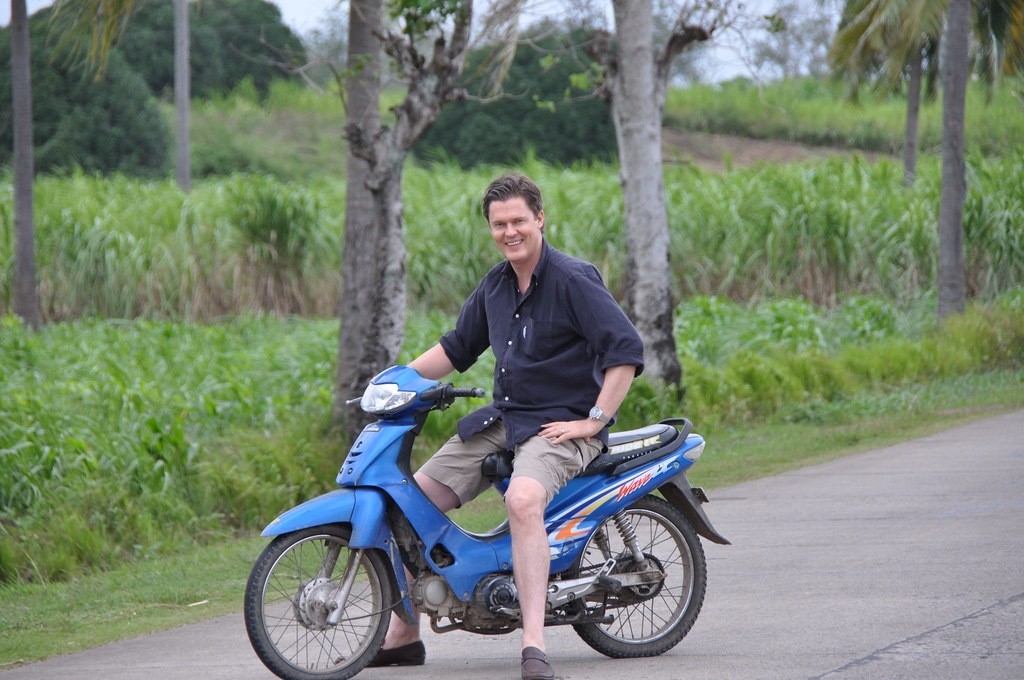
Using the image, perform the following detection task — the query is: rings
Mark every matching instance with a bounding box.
[560,429,564,433]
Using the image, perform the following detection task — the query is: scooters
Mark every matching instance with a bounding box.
[243,363,734,680]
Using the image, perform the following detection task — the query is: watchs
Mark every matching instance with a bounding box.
[589,407,610,423]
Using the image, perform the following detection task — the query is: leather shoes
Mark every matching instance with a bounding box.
[334,639,426,668]
[521,646,555,680]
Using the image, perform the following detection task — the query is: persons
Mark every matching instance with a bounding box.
[334,175,645,680]
